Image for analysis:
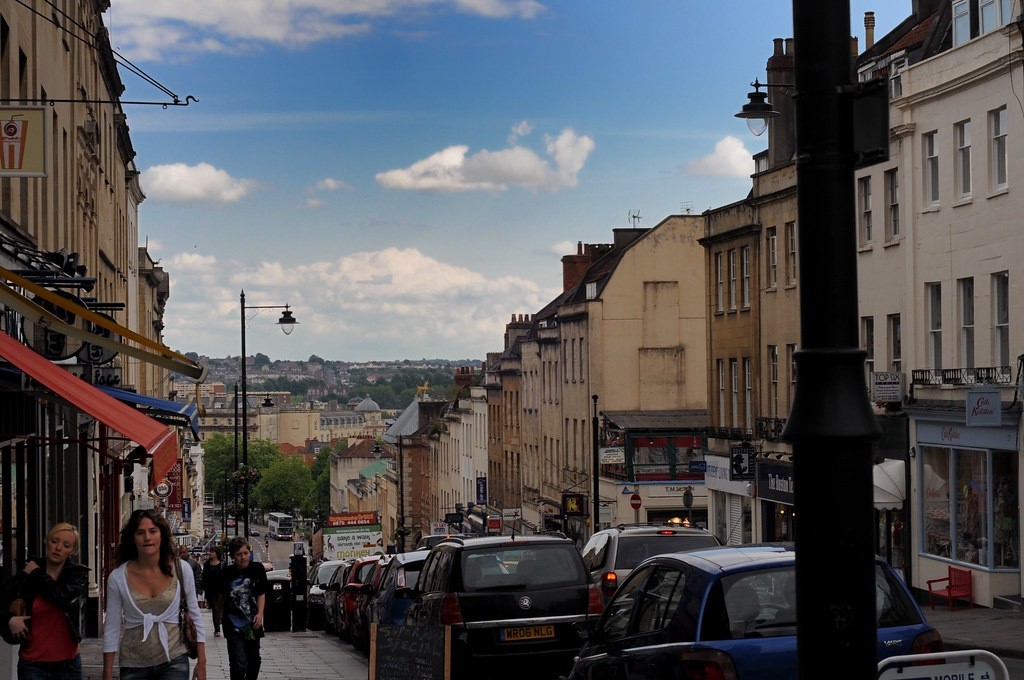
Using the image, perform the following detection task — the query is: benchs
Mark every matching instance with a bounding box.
[927,565,974,608]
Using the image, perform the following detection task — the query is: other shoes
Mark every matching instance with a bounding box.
[214,631,221,636]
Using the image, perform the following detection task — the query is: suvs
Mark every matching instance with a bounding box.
[580,523,725,608]
[396,531,604,680]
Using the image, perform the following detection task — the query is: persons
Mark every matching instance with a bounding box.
[293,526,305,541]
[202,546,228,637]
[215,538,270,680]
[179,545,203,600]
[687,444,698,457]
[955,485,978,548]
[102,509,207,680]
[0,522,93,680]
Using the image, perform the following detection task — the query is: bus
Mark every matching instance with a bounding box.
[415,535,471,552]
[267,512,294,540]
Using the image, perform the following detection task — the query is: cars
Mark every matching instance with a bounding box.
[223,516,261,537]
[261,549,432,661]
[561,541,946,680]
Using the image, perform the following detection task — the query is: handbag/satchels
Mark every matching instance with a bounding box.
[2,559,32,645]
[174,555,198,660]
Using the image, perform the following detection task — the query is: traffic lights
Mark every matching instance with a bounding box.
[266,541,268,548]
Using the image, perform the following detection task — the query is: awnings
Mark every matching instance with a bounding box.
[0,329,203,507]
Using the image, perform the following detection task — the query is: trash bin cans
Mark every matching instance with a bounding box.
[264,578,292,631]
[85,582,101,638]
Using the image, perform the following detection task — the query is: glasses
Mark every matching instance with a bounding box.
[133,509,159,516]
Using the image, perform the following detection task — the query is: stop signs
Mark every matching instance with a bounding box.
[630,494,641,510]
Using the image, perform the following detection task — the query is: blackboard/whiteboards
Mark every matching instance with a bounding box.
[369,623,452,680]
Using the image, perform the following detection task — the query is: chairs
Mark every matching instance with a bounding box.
[516,559,547,585]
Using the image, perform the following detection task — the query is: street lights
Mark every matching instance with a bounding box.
[372,440,405,529]
[232,383,276,535]
[592,393,602,531]
[240,290,299,543]
